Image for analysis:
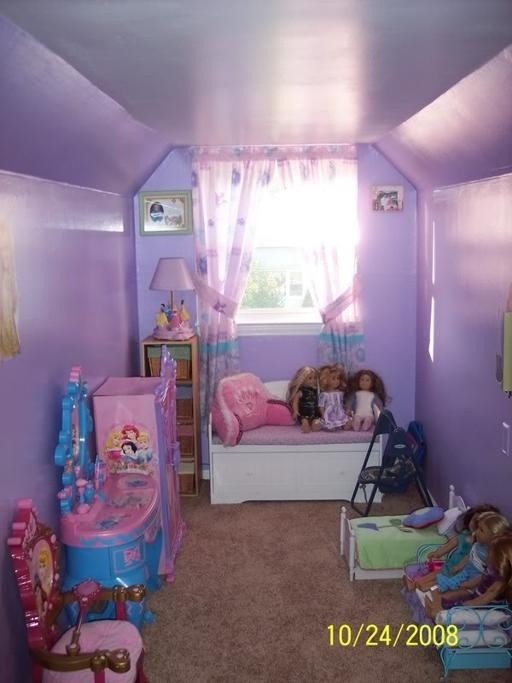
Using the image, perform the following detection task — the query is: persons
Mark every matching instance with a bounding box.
[317,364,354,432]
[435,511,509,591]
[284,364,321,432]
[400,503,498,589]
[341,368,387,431]
[424,536,511,618]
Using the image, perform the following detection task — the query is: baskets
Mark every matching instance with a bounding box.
[147,347,190,378]
[177,461,194,495]
[174,387,192,421]
[178,425,194,457]
